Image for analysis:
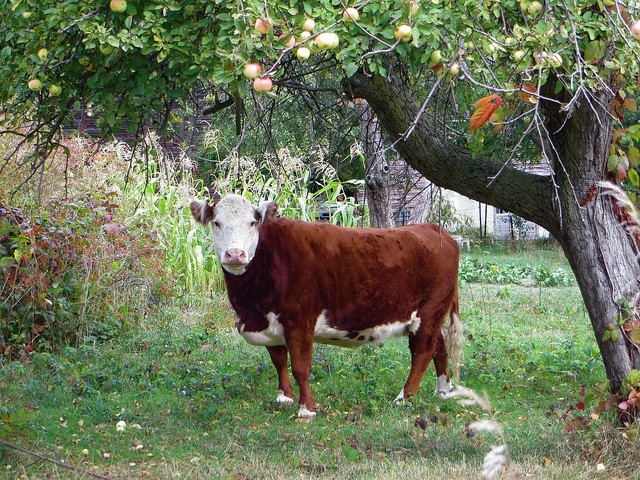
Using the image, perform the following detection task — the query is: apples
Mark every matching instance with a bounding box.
[343,8,359,23]
[399,24,413,42]
[316,32,333,50]
[22,10,32,21]
[255,18,274,34]
[513,50,525,62]
[38,47,50,63]
[298,47,310,61]
[100,44,113,54]
[467,42,474,49]
[551,52,563,67]
[406,1,420,20]
[79,54,90,67]
[72,46,81,58]
[487,44,498,53]
[278,35,297,48]
[295,31,310,43]
[427,58,436,68]
[116,419,126,427]
[630,21,640,40]
[393,29,400,41]
[504,37,519,51]
[331,33,340,51]
[534,53,546,66]
[254,78,274,93]
[109,0,128,12]
[49,84,64,96]
[528,2,542,15]
[28,79,41,92]
[431,50,444,62]
[449,61,461,75]
[302,18,317,31]
[244,63,262,78]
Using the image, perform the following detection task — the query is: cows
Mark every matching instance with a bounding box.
[190,194,462,423]
